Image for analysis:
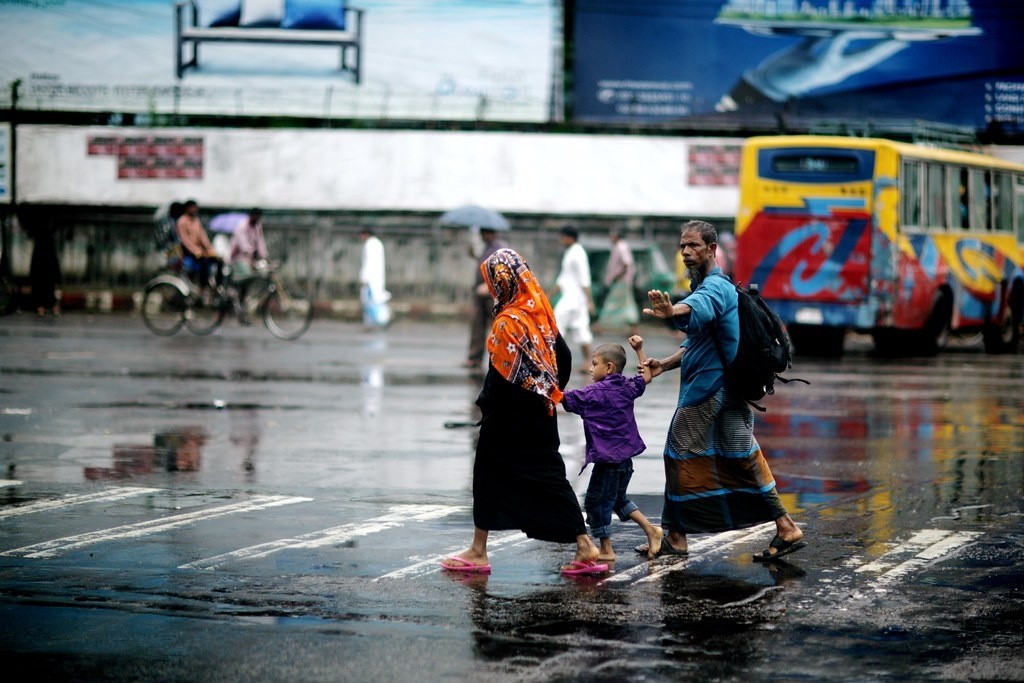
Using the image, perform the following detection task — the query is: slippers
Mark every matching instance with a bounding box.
[751,536,809,562]
[634,536,691,556]
[560,562,610,574]
[440,556,493,574]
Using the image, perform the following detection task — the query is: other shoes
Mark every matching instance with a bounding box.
[460,360,481,370]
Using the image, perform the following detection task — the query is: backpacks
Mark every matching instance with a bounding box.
[704,273,793,405]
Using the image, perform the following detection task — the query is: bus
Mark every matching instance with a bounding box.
[734,136,1024,358]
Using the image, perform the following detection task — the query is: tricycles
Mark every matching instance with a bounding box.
[140,260,312,341]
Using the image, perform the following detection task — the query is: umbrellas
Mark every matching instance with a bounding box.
[437,205,508,229]
[209,212,250,234]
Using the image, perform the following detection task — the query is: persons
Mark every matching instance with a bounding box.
[463,228,508,367]
[546,225,598,374]
[440,248,609,576]
[560,334,663,560]
[356,229,387,332]
[590,228,639,337]
[635,220,810,561]
[175,200,272,323]
[673,230,736,295]
